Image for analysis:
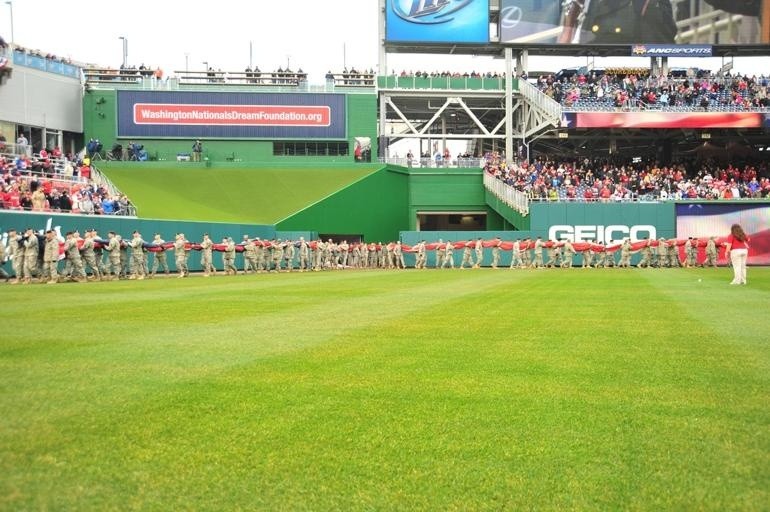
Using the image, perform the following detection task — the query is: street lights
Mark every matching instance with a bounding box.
[184,53,191,79]
[117,35,128,68]
[201,60,210,82]
[4,0,15,42]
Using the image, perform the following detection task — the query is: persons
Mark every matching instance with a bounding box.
[558,0,678,45]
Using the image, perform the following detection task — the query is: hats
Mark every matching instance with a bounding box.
[5,227,226,239]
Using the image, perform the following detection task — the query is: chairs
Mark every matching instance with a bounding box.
[487,154,684,202]
[534,75,770,112]
[0,156,133,217]
